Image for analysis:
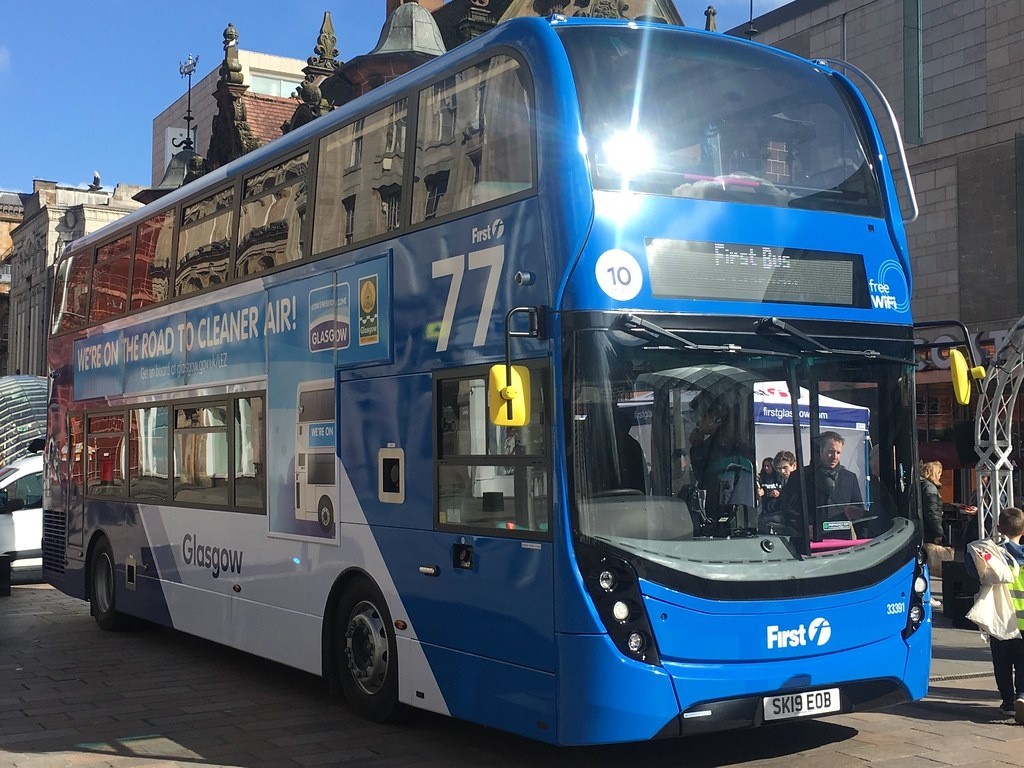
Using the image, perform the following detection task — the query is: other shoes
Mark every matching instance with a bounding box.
[931,597,940,607]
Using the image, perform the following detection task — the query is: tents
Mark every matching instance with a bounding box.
[611,381,877,434]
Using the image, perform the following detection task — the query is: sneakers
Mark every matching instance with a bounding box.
[999,701,1015,716]
[1015,692,1024,725]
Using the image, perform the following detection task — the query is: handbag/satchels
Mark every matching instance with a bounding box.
[966,535,1023,644]
[923,540,944,577]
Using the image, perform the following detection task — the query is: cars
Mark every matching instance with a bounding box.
[0,453,44,559]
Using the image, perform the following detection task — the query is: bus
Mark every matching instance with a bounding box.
[41,15,985,744]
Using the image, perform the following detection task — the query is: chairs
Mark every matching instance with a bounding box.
[566,408,648,539]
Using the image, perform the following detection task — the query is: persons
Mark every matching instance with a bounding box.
[774,432,864,540]
[755,457,785,515]
[613,410,646,495]
[966,506,1024,726]
[909,461,979,612]
[687,388,730,489]
[951,464,1010,600]
[844,444,892,537]
[773,449,796,479]
